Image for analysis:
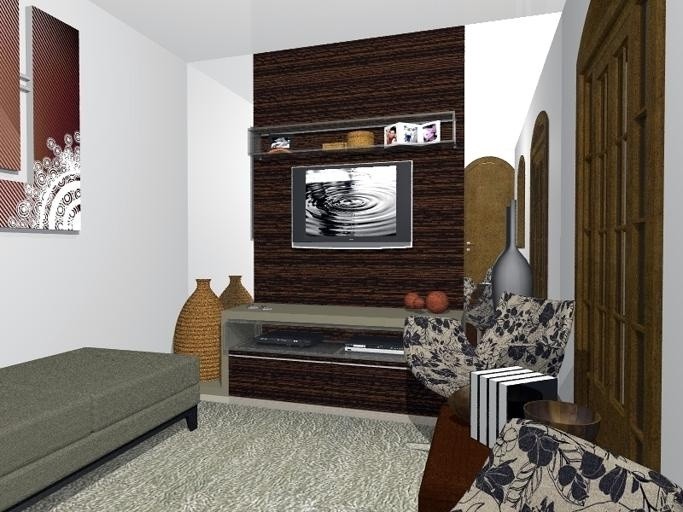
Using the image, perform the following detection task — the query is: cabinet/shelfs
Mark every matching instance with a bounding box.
[247,110,457,162]
[220,301,467,418]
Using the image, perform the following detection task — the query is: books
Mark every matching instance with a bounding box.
[469,366,557,451]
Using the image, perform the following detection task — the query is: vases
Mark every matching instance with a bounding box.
[489,195,535,318]
[218,275,254,311]
[172,277,224,384]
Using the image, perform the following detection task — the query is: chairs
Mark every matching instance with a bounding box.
[445,416,681,512]
[399,291,575,447]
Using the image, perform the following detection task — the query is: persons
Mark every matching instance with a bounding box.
[386,126,397,144]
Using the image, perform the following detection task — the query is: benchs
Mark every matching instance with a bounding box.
[0,345,201,511]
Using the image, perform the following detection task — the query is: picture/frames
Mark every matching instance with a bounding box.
[381,118,441,147]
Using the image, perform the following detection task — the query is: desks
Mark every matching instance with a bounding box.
[414,366,639,511]
